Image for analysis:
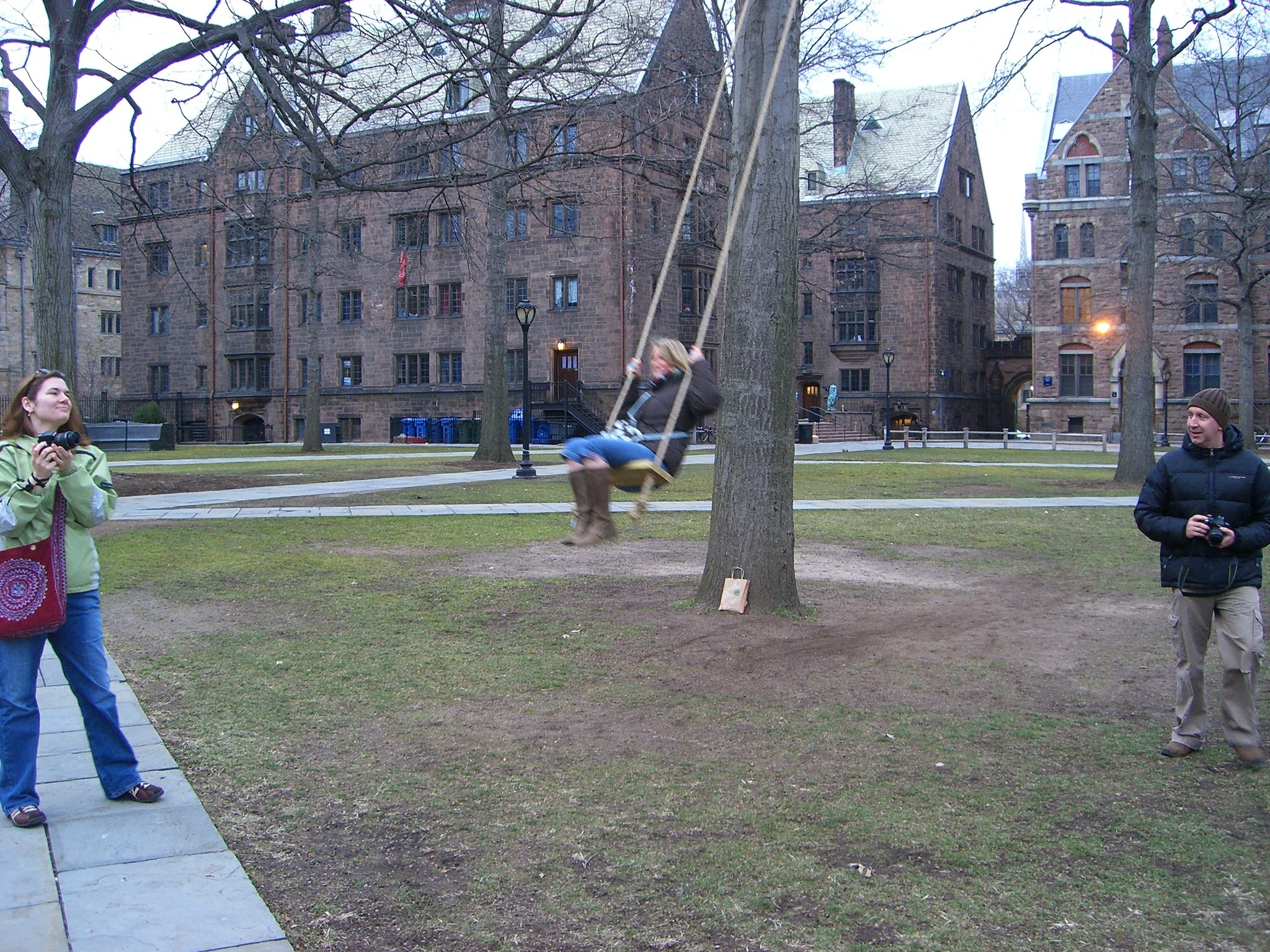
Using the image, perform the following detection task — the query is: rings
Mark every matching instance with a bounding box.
[629,363,636,369]
[45,457,50,462]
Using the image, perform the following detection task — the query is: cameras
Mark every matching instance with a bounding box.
[36,430,80,463]
[1198,515,1232,546]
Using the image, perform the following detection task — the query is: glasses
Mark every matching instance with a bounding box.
[25,368,66,396]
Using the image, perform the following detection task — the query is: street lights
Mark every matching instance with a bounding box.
[511,299,541,479]
[1117,368,1124,431]
[1160,355,1172,447]
[882,348,895,450]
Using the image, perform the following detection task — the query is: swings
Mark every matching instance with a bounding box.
[597,0,800,488]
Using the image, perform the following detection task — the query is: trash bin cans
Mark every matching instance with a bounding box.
[521,416,537,442]
[508,408,523,444]
[472,418,482,443]
[440,417,458,443]
[910,424,929,440]
[320,422,342,443]
[799,424,813,443]
[457,418,473,443]
[428,418,443,443]
[566,422,582,439]
[400,418,416,437]
[389,417,403,443]
[808,407,820,422]
[415,418,428,438]
[548,420,565,444]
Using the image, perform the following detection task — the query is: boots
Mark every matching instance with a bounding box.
[561,469,591,546]
[573,467,617,546]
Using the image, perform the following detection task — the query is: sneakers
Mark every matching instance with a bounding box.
[1230,746,1265,765]
[7,805,47,827]
[1161,742,1192,757]
[108,779,164,803]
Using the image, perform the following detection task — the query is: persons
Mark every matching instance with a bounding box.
[1134,387,1270,769]
[559,334,721,548]
[0,369,165,829]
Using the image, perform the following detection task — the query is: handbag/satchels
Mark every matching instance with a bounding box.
[718,566,750,614]
[600,419,645,443]
[0,537,67,641]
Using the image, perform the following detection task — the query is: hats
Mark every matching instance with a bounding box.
[1187,388,1231,434]
